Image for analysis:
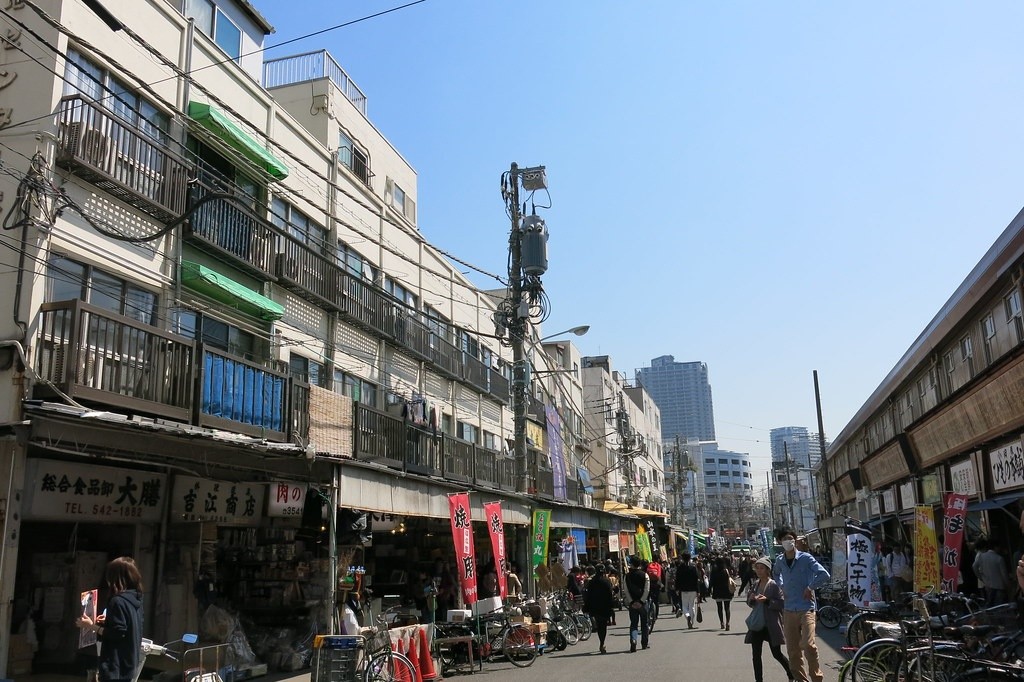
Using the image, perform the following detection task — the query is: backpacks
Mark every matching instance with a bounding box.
[647,561,661,581]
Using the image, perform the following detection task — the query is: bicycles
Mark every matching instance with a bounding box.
[644,592,657,635]
[436,588,593,670]
[814,583,1024,682]
[361,604,417,682]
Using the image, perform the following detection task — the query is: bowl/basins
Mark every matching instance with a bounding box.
[869,602,885,610]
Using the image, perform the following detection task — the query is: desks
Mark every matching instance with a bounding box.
[433,634,480,675]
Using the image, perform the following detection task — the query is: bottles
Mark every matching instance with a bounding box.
[626,556,630,561]
[355,566,366,592]
[346,566,355,578]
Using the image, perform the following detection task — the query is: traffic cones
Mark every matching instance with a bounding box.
[408,637,423,682]
[389,643,402,682]
[397,639,411,682]
[418,627,438,682]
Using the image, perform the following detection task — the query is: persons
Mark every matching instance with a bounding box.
[771,526,831,682]
[709,557,734,631]
[661,537,767,579]
[1015,508,1024,594]
[675,553,700,629]
[696,562,712,603]
[737,555,760,596]
[873,535,1024,608]
[744,556,795,682]
[76,556,144,682]
[342,591,391,681]
[667,560,683,618]
[410,556,524,623]
[566,554,625,625]
[583,562,622,654]
[806,546,832,558]
[623,556,650,652]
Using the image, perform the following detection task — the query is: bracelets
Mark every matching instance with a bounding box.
[807,586,812,591]
[97,626,101,633]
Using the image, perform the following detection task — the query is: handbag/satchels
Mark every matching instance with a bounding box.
[697,605,702,623]
[725,568,737,594]
[703,575,709,588]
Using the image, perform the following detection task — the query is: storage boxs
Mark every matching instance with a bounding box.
[6,632,34,678]
[532,632,547,644]
[529,622,547,634]
[471,596,501,616]
[447,609,472,623]
[509,616,532,625]
[312,637,357,649]
[311,648,358,682]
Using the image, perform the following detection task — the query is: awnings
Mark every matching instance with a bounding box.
[963,490,1024,537]
[868,515,895,527]
[188,101,289,184]
[181,262,286,320]
[26,392,642,636]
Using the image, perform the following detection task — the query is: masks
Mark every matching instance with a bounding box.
[782,540,795,552]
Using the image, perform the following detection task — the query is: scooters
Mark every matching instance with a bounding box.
[93,607,199,682]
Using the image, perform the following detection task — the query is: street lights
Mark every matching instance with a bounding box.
[513,324,592,599]
[799,466,833,555]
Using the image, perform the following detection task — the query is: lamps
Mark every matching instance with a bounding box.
[371,514,379,521]
[400,518,407,527]
[389,514,394,521]
[381,513,385,521]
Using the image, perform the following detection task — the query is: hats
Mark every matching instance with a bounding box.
[568,566,581,572]
[755,556,772,573]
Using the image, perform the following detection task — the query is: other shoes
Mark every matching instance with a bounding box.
[725,623,730,631]
[687,616,692,628]
[630,639,637,653]
[721,622,724,629]
[600,645,606,653]
[642,645,650,649]
[676,609,682,618]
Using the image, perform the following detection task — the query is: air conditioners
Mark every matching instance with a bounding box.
[384,315,407,343]
[274,253,300,282]
[64,121,119,176]
[51,344,103,391]
[578,493,592,508]
